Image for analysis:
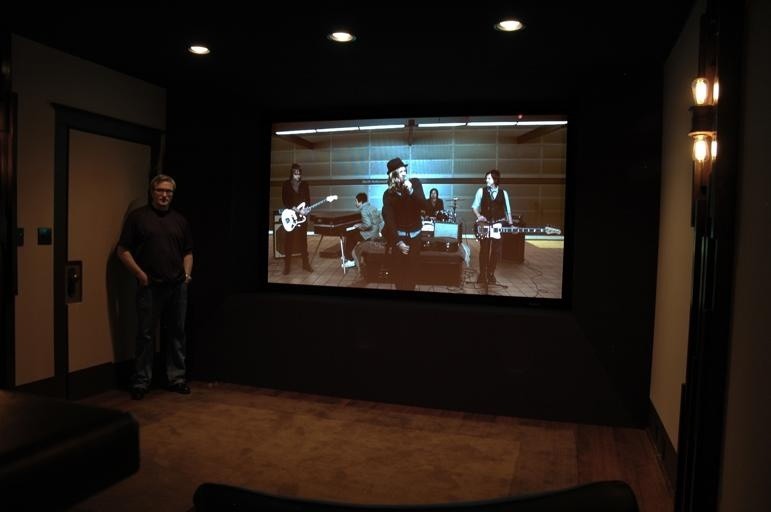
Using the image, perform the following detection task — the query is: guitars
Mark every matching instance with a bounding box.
[281,195,338,232]
[474,219,561,240]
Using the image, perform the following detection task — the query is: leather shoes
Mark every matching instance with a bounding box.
[477,273,485,283]
[133,387,146,400]
[166,383,191,394]
[487,272,496,283]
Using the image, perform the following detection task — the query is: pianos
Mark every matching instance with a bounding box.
[310,210,361,236]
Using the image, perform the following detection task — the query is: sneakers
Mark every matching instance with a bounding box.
[341,260,356,268]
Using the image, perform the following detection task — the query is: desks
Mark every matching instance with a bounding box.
[1,384,143,511]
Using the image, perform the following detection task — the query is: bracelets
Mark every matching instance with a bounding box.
[185,272,192,280]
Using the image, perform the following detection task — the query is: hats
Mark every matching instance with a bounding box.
[386,158,407,175]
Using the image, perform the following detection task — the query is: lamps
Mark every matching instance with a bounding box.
[687,76,715,166]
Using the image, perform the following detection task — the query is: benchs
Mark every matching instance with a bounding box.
[186,480,645,512]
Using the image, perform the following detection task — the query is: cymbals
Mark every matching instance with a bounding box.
[450,204,462,209]
[445,197,466,202]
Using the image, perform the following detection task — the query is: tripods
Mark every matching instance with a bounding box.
[466,208,508,295]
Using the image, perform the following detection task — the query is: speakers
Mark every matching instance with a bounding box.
[273,210,308,259]
[500,231,526,266]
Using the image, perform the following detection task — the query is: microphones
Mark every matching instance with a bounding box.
[403,176,408,190]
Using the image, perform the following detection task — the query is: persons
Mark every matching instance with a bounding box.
[115,174,196,399]
[381,157,427,291]
[340,193,380,270]
[423,188,444,221]
[472,169,513,284]
[281,163,314,276]
